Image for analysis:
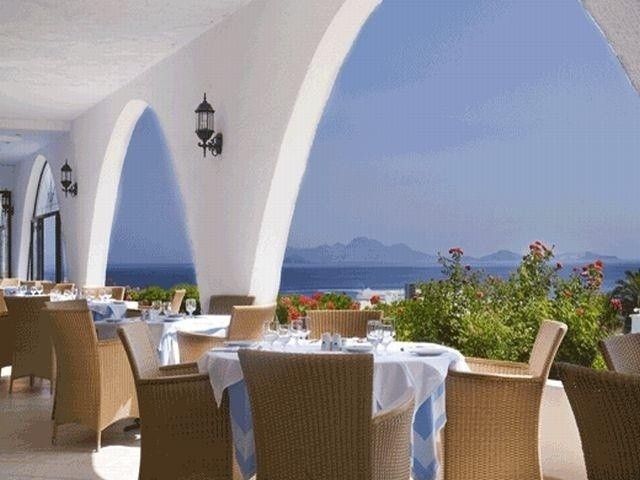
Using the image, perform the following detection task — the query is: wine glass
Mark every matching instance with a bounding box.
[291,317,310,350]
[16,285,26,295]
[163,302,171,317]
[98,287,113,302]
[186,299,197,318]
[263,322,277,351]
[36,285,44,296]
[379,325,396,355]
[30,287,36,296]
[152,300,161,315]
[278,325,290,351]
[366,320,383,356]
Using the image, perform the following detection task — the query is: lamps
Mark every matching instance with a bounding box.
[194,93,222,157]
[61,158,78,197]
[2,188,14,215]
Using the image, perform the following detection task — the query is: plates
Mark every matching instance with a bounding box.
[410,350,447,357]
[346,345,372,352]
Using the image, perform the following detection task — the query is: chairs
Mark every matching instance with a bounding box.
[53,284,75,294]
[111,287,125,301]
[305,310,384,340]
[558,362,640,480]
[177,303,275,364]
[0,311,12,377]
[116,320,233,480]
[41,307,140,452]
[0,289,8,312]
[237,348,416,480]
[445,319,568,480]
[598,332,640,371]
[207,295,256,315]
[141,289,187,313]
[4,296,57,395]
[43,299,89,310]
